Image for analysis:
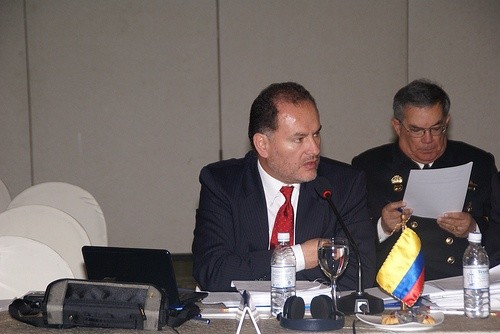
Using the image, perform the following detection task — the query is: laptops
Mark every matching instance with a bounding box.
[80,246,208,310]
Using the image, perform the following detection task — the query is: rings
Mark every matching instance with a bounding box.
[453,226,458,232]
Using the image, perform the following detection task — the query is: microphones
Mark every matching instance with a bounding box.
[311,177,381,314]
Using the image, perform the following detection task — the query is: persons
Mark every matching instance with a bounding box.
[352,78,500,280]
[191,82,378,291]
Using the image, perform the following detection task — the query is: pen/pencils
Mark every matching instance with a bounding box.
[385,199,403,213]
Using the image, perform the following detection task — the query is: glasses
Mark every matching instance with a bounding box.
[400,119,446,138]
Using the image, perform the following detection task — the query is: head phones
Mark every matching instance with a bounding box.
[276,296,345,331]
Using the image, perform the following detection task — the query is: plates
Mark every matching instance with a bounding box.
[355,313,445,332]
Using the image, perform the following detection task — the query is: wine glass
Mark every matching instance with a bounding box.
[318,237,350,311]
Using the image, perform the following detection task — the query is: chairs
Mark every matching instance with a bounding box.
[0,181,107,299]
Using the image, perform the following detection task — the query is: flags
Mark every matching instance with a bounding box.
[375,227,424,307]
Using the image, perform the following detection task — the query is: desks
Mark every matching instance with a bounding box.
[0,290,500,333]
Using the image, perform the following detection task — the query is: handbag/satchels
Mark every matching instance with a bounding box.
[9,278,168,330]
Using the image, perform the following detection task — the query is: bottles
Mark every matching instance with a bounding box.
[271,232,297,317]
[462,231,490,319]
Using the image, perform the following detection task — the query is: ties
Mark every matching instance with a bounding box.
[422,164,430,170]
[270,186,294,250]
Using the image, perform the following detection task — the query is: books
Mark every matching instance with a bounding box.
[199,264,334,319]
[340,264,500,316]
